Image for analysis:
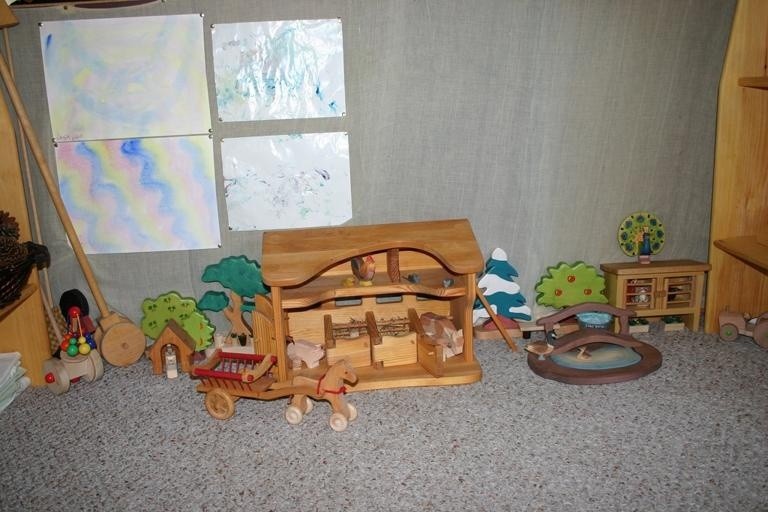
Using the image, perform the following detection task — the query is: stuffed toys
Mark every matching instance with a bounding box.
[0,210,51,310]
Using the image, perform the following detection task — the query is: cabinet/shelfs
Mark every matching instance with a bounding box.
[249,217,486,393]
[0,83,58,397]
[601,257,711,334]
[700,1,768,338]
